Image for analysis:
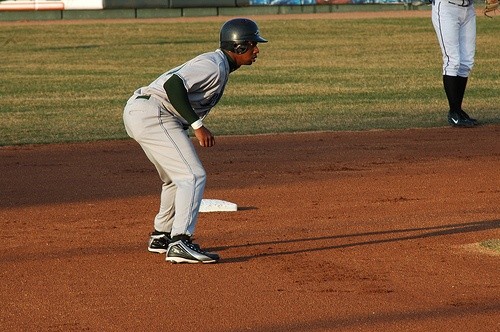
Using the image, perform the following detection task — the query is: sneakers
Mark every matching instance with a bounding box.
[165,238,220,263]
[448,110,474,128]
[459,109,478,125]
[148,232,173,253]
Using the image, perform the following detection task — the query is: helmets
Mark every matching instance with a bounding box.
[219,18,268,43]
